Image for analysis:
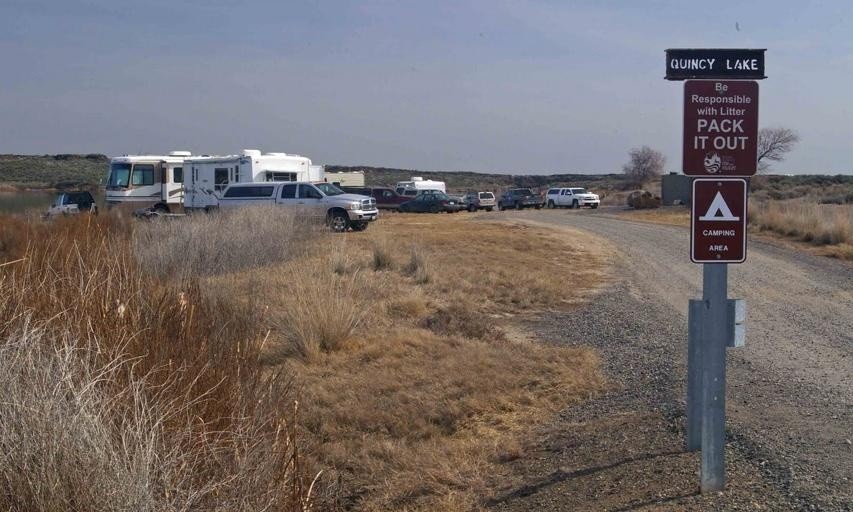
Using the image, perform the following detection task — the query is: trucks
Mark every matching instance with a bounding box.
[211,179,379,234]
[102,150,191,214]
[543,187,601,210]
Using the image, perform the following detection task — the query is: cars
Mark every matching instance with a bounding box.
[396,186,496,214]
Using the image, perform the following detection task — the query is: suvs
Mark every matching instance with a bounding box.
[40,187,99,219]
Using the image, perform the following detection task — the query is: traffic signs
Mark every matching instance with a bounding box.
[663,45,773,84]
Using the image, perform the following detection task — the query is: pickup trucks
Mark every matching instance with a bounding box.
[496,187,540,212]
[342,184,414,210]
